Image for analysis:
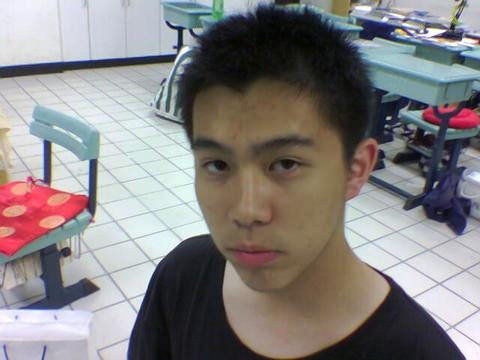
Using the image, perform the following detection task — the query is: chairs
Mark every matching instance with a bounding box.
[0,105,100,310]
[390,62,480,179]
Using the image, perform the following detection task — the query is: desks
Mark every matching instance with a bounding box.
[395,33,472,66]
[459,47,480,71]
[336,38,480,211]
[159,3,216,57]
[287,5,363,42]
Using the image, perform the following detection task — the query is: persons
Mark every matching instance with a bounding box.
[126,4,469,359]
